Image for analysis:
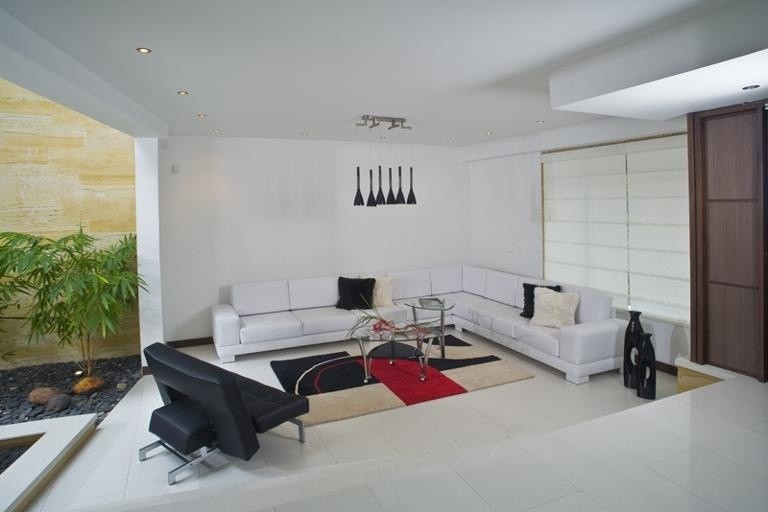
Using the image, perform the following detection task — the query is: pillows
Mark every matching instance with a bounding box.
[336,276,376,309]
[519,282,560,318]
[529,287,580,330]
[371,277,396,307]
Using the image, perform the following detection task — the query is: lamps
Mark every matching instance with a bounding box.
[353,114,417,207]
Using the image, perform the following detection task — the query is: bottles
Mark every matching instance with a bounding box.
[635,333,656,400]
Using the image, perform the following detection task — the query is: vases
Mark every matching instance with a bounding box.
[638,331,657,400]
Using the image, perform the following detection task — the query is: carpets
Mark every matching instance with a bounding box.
[269,333,534,426]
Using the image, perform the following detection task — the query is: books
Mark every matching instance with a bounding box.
[418,298,444,308]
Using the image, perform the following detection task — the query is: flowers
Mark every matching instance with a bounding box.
[342,291,437,344]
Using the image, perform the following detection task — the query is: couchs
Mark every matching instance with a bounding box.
[209,260,626,388]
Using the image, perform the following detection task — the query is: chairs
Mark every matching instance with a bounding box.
[138,341,309,487]
